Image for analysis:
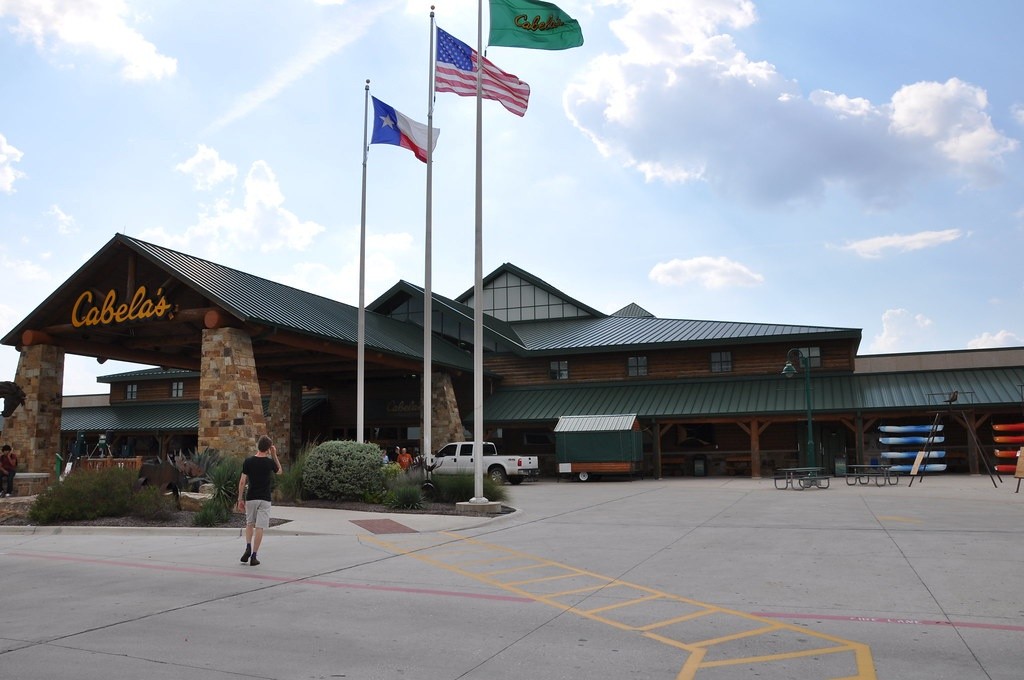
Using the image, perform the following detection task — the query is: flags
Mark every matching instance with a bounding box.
[434,24,530,116]
[369,95,440,163]
[488,0,584,51]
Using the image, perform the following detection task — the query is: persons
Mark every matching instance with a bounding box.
[0,444,18,497]
[382,449,389,462]
[237,434,282,567]
[393,446,401,461]
[397,446,420,471]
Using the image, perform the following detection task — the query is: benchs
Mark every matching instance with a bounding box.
[771,475,833,489]
[843,472,902,486]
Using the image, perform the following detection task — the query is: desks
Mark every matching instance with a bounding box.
[777,467,823,489]
[848,464,892,486]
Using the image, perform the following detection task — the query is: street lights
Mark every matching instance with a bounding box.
[780,347,820,487]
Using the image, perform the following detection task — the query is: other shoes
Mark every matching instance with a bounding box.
[240,548,251,562]
[0,489,5,498]
[250,558,260,566]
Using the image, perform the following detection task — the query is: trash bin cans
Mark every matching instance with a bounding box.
[694,453,707,477]
[835,453,847,477]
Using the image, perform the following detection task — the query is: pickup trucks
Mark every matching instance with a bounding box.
[414,441,538,486]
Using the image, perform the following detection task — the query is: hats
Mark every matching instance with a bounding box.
[395,445,400,449]
